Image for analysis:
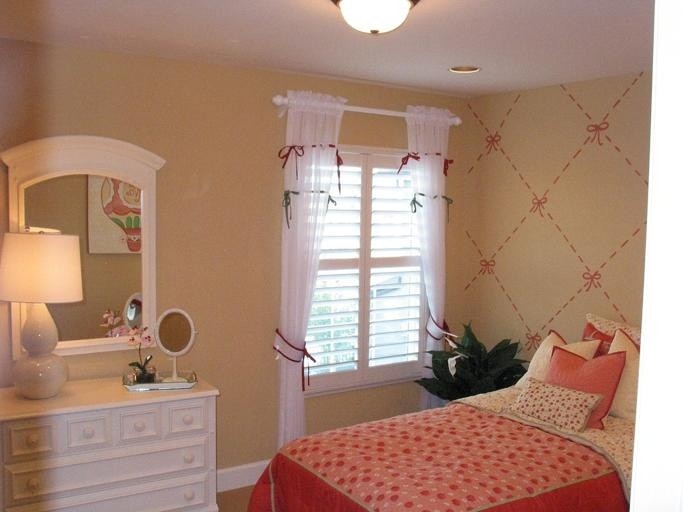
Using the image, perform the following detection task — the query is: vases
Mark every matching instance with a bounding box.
[126,367,157,383]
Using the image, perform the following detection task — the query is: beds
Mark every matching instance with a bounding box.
[235,320,632,508]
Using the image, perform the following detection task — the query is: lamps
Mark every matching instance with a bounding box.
[327,0,422,34]
[0,232,84,400]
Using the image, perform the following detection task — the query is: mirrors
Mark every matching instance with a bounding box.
[122,291,144,332]
[2,131,167,361]
[154,306,196,384]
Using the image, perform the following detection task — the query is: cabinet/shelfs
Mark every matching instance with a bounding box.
[0,374,221,511]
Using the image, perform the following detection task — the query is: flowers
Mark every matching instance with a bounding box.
[96,305,128,339]
[409,322,529,402]
[123,325,156,378]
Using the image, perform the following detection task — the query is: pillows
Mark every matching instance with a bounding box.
[602,324,636,424]
[547,342,624,434]
[586,312,640,345]
[581,322,613,358]
[516,329,601,390]
[519,378,603,434]
